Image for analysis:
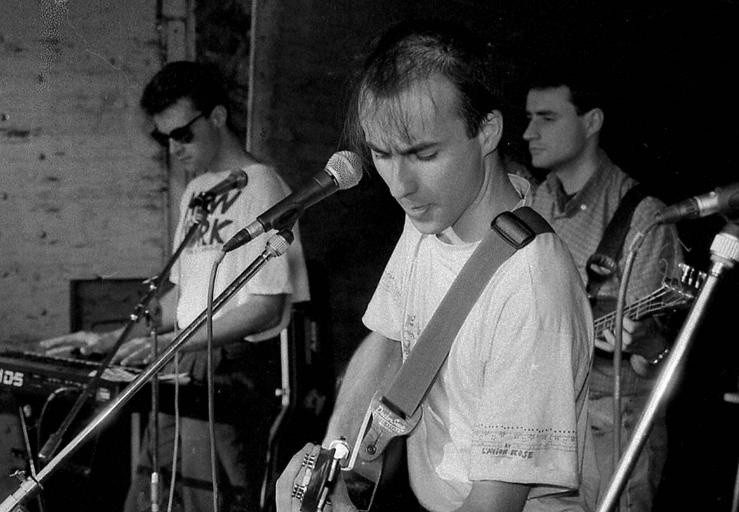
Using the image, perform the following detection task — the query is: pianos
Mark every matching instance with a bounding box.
[0,348,267,429]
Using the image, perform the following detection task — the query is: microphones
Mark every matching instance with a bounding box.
[222,151,363,253]
[655,185,739,224]
[189,169,249,208]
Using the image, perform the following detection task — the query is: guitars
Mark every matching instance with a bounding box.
[290,445,384,511]
[594,261,705,340]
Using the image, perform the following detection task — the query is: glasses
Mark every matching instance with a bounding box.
[150,115,203,146]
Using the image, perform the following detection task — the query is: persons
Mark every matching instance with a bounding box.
[521,57,687,511]
[41,55,312,511]
[318,33,598,511]
